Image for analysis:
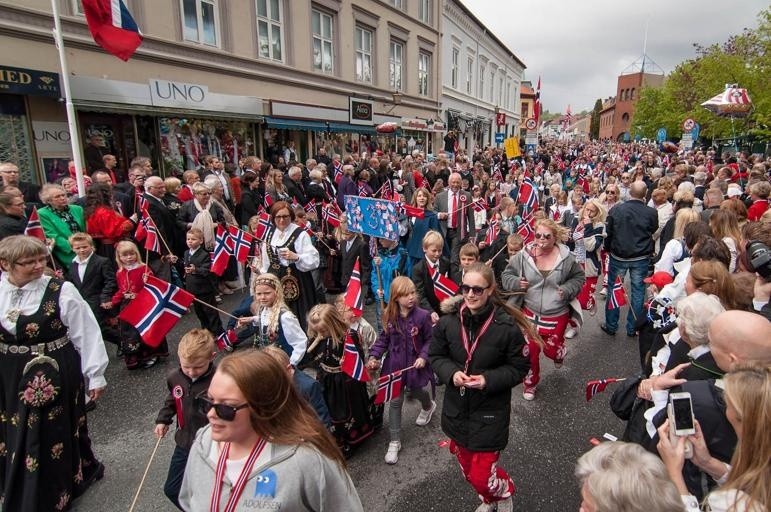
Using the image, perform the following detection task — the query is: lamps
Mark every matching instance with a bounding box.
[488,104,500,114]
[383,89,403,107]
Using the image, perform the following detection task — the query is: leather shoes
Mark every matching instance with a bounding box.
[143,355,160,370]
[626,329,637,338]
[600,322,616,336]
[117,344,125,358]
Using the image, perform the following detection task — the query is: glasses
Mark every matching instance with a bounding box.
[3,255,49,270]
[534,232,555,240]
[458,282,493,297]
[190,386,249,423]
[275,215,292,220]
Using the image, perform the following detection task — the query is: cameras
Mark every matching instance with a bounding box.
[135,175,149,184]
[745,239,771,282]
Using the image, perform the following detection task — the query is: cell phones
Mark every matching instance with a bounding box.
[669,392,695,436]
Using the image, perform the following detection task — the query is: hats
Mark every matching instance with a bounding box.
[677,181,696,193]
[643,270,673,289]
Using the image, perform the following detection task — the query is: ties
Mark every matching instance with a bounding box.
[451,192,458,229]
[432,265,440,271]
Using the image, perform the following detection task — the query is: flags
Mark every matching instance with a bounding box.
[563,106,571,130]
[535,76,540,121]
[81,0,143,62]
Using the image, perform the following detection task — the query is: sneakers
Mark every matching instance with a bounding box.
[588,294,597,318]
[599,286,608,297]
[521,385,537,402]
[564,326,579,340]
[554,358,566,370]
[497,496,514,512]
[474,501,497,512]
[415,400,437,427]
[381,440,406,465]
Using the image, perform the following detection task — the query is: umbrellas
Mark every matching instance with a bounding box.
[700,84,755,130]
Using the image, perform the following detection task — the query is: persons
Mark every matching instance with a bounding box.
[1,131,770,511]
